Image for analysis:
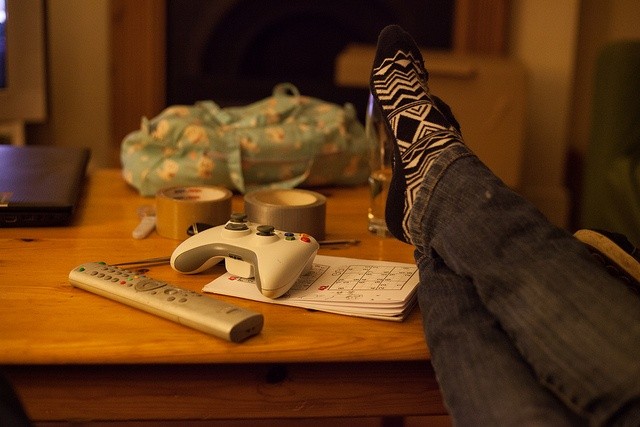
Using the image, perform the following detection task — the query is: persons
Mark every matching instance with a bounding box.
[369,26,640,427]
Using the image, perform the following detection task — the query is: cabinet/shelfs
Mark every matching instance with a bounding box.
[47,0,167,163]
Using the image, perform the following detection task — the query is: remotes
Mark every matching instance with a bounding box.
[68,262,264,344]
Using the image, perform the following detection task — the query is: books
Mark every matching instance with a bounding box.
[202,254,421,321]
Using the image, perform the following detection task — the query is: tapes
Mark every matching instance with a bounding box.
[155,183,233,241]
[243,189,327,242]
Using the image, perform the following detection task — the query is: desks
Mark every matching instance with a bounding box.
[0,164,448,427]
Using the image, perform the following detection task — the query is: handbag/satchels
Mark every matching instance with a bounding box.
[120,83,383,196]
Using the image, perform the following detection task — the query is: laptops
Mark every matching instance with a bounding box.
[0,144,90,227]
